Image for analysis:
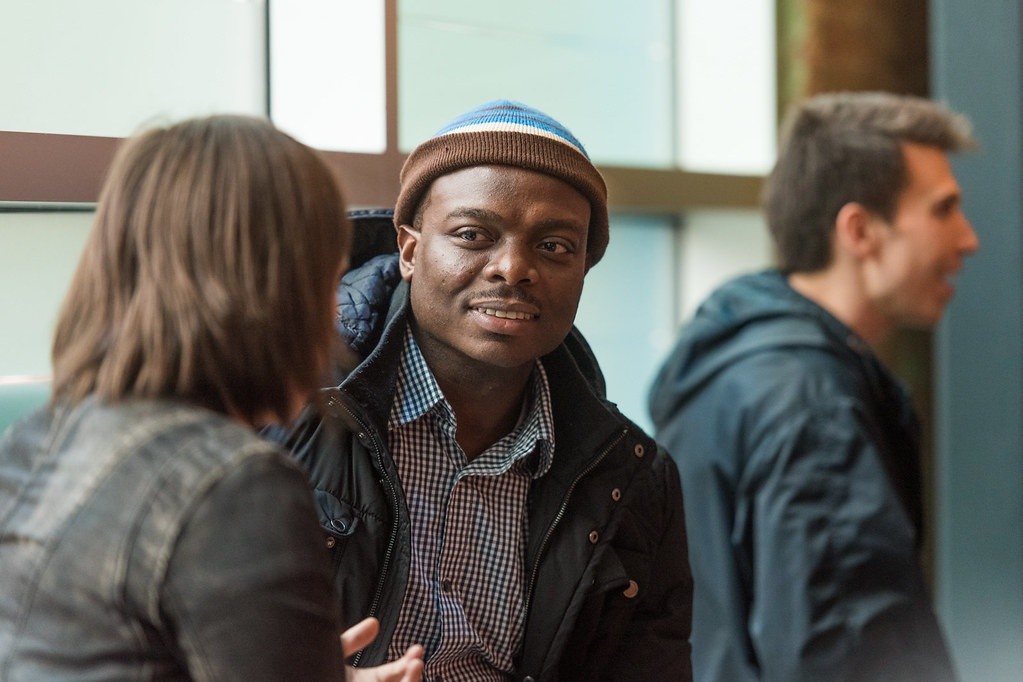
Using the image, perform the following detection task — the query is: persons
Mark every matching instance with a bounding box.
[649,90,980,682]
[0,112,428,682]
[260,99,696,682]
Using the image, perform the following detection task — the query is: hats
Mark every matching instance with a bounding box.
[390,97,611,266]
